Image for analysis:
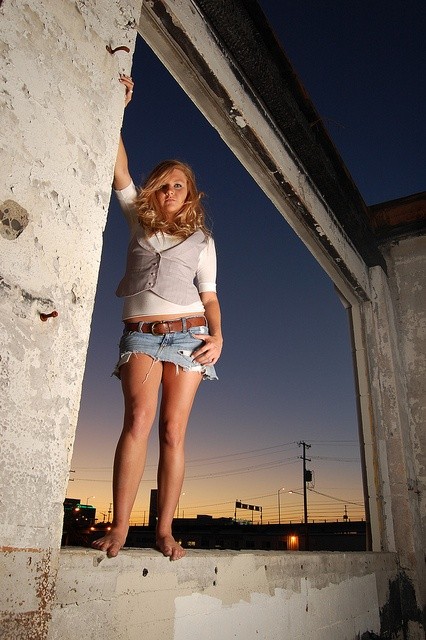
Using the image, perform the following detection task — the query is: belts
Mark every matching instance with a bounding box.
[124,317,206,336]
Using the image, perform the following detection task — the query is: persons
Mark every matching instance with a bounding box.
[91,72,224,562]
